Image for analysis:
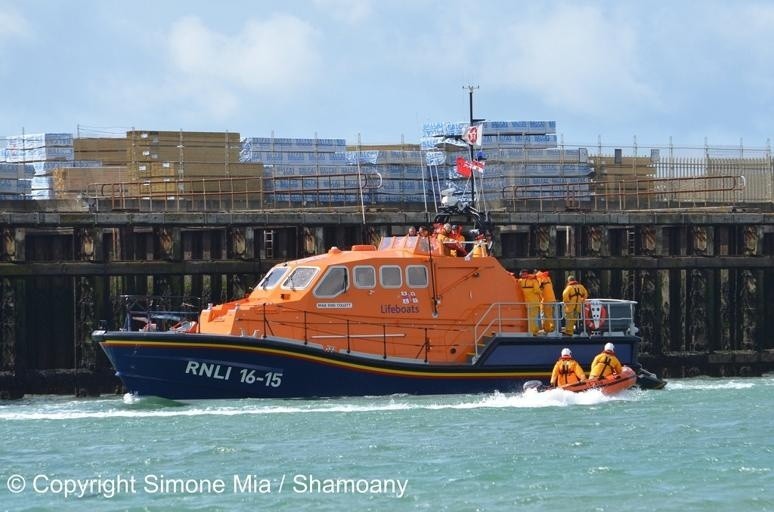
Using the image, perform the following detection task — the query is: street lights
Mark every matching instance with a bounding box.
[439,84,489,206]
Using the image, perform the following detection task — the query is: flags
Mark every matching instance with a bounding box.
[419,120,488,205]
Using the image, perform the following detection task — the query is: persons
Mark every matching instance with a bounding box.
[399,221,495,257]
[560,275,590,336]
[532,267,557,332]
[516,268,543,336]
[588,342,623,380]
[549,348,591,389]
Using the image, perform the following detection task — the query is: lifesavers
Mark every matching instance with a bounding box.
[585,303,606,329]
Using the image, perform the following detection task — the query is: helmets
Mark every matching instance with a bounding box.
[604,342,614,352]
[561,348,572,358]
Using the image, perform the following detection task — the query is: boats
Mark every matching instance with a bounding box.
[522,364,638,396]
[90,214,667,399]
